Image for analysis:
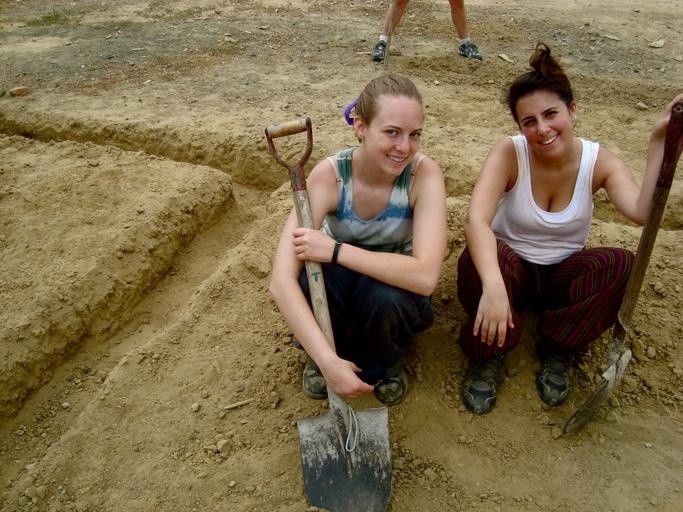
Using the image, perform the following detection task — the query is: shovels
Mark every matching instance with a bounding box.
[562,100,683,440]
[265,117,392,512]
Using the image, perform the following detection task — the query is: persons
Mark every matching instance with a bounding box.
[371,0,483,64]
[452,40,683,416]
[268,75,447,407]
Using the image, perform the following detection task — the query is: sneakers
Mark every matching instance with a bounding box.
[371,355,409,407]
[300,359,330,399]
[456,39,485,61]
[459,350,505,415]
[536,342,574,407]
[369,38,391,62]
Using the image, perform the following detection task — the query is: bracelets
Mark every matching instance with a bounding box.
[330,238,343,266]
